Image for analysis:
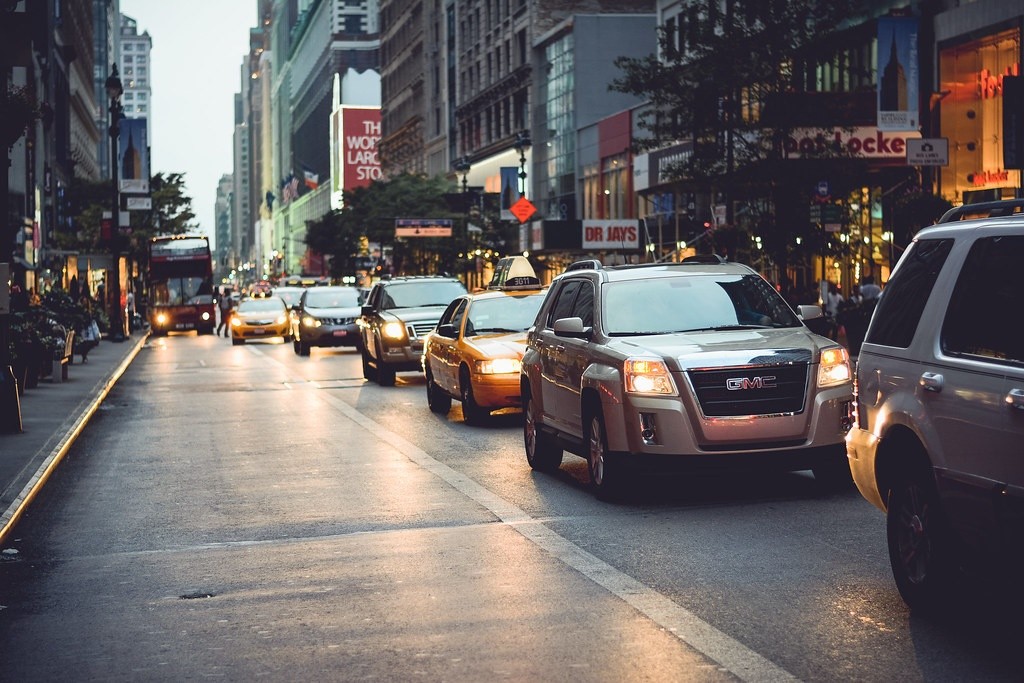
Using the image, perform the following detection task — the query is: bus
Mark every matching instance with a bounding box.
[146,234,217,336]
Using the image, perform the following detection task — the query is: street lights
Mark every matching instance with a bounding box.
[102,62,129,341]
[513,134,533,198]
[455,162,471,260]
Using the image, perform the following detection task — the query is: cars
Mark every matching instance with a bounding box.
[421,283,551,426]
[547,380,580,414]
[230,296,295,346]
[266,287,307,312]
[291,286,367,357]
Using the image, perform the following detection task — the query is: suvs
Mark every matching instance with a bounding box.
[843,199,1024,620]
[519,253,854,507]
[358,273,470,387]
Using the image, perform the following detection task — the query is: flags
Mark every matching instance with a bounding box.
[266,171,319,212]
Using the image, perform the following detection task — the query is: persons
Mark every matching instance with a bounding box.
[822,276,883,341]
[212,284,232,338]
[96,275,135,336]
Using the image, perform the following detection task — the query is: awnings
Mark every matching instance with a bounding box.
[13,256,35,272]
